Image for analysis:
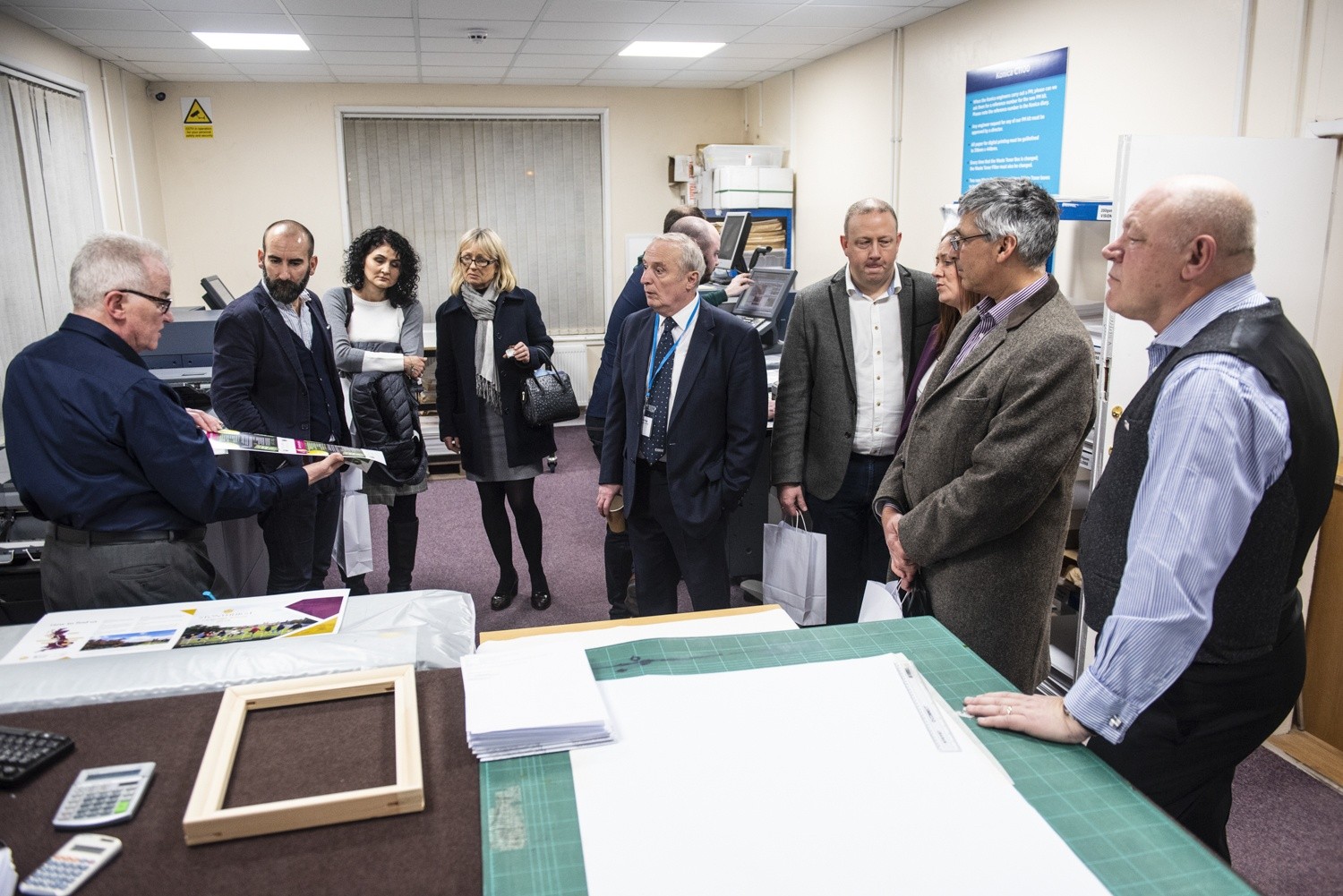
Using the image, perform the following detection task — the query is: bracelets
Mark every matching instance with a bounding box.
[406,355,414,379]
[1062,704,1094,733]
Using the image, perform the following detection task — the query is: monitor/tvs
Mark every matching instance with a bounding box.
[714,211,753,270]
[732,268,798,320]
[200,275,236,310]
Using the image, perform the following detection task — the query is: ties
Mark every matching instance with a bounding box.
[640,316,678,467]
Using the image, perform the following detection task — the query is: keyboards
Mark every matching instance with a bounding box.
[0,724,77,788]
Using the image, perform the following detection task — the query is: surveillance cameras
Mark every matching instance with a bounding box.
[147,86,166,102]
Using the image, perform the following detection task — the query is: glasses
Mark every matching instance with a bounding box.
[950,233,993,250]
[459,252,498,268]
[104,289,172,315]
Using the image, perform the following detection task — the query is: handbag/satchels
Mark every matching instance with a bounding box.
[763,508,828,625]
[522,346,579,425]
[857,578,914,622]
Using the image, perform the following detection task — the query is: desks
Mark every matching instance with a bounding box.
[0,616,1264,896]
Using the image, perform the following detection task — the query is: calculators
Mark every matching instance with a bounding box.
[50,761,156,828]
[19,834,124,896]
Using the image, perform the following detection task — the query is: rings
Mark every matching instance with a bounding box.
[780,506,785,509]
[419,373,423,377]
[1008,705,1013,714]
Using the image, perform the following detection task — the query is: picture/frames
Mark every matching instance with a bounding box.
[180,663,426,850]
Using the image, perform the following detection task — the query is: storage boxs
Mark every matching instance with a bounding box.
[667,142,794,209]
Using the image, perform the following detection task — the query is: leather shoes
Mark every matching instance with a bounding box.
[491,571,518,610]
[529,581,551,611]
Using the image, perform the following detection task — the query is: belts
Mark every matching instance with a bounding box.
[47,523,207,545]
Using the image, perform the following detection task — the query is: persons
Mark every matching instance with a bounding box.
[772,198,940,630]
[3,233,344,612]
[321,226,427,596]
[586,205,767,620]
[963,174,1335,867]
[210,219,353,596]
[873,178,1097,696]
[435,227,556,610]
